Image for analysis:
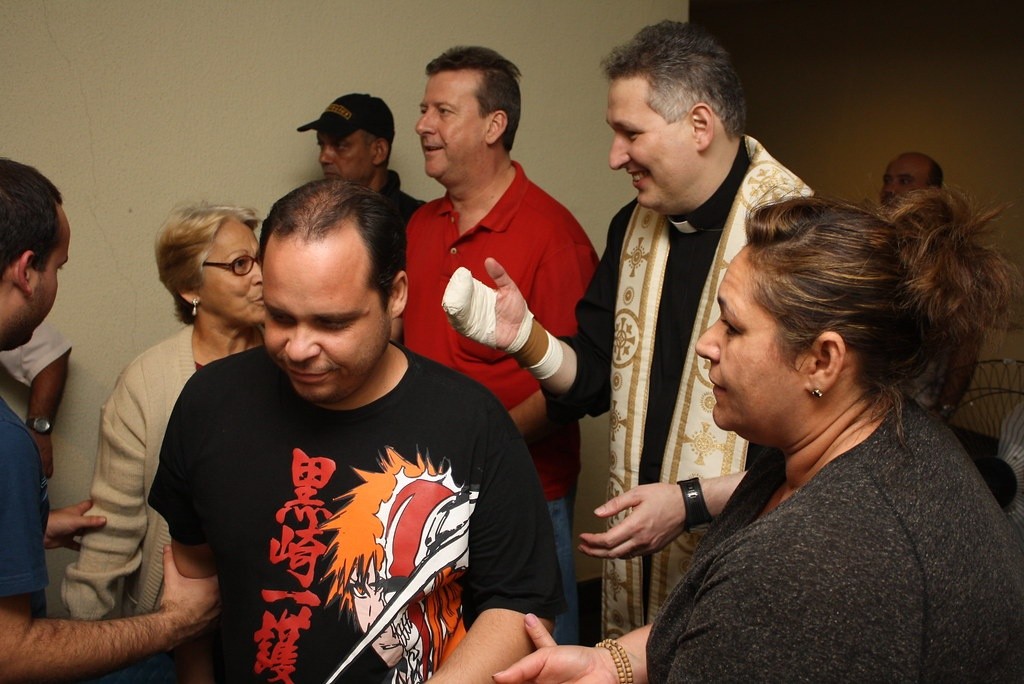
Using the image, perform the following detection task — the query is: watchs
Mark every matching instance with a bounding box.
[933,401,955,417]
[677,478,714,534]
[25,417,54,434]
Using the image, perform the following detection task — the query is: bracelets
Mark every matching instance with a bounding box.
[594,639,633,684]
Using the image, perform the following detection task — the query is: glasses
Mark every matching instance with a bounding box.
[203,255,262,276]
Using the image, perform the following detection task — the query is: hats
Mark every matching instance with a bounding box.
[296,93,395,144]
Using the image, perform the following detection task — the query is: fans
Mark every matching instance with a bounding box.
[948,358,1024,537]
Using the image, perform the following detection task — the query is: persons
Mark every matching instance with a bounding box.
[58,200,267,622]
[392,44,599,650]
[0,158,222,684]
[148,176,564,684]
[880,151,985,427]
[1,302,72,477]
[443,10,817,641]
[490,191,1022,684]
[256,94,428,253]
